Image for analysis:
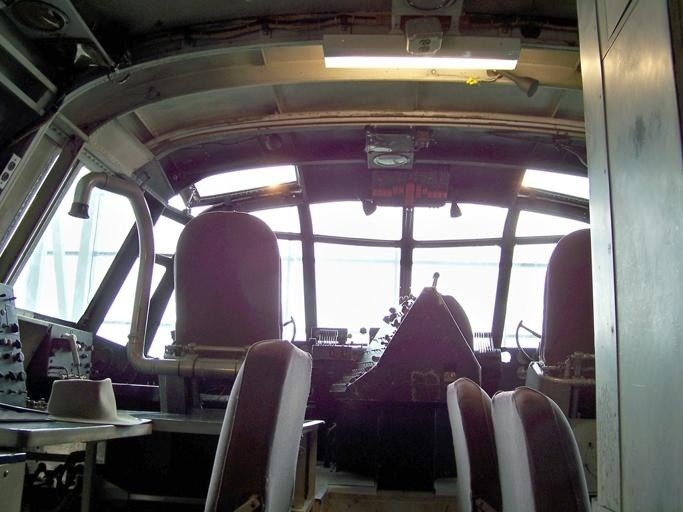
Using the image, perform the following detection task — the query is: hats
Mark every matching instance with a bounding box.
[48,377,152,426]
